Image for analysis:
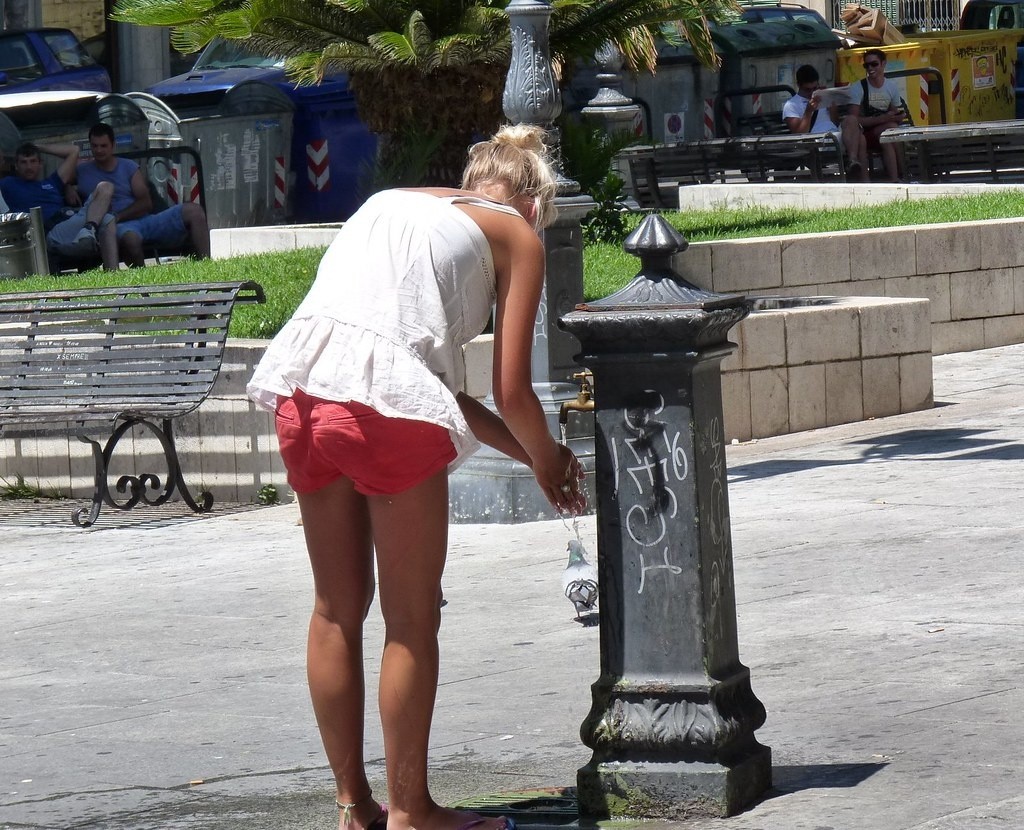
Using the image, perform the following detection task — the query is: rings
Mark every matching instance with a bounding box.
[560,484,570,492]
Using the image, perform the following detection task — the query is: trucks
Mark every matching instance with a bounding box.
[960,0,1024,97]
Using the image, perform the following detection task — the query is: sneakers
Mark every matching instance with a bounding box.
[71,226,98,254]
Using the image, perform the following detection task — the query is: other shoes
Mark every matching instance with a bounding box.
[848,164,861,182]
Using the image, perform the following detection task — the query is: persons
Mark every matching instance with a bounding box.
[0,143,120,273]
[848,49,919,184]
[782,65,870,182]
[63,123,210,268]
[245,123,587,830]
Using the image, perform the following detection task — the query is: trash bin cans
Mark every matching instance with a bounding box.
[902,28,1024,124]
[836,39,940,127]
[272,72,378,223]
[618,36,726,145]
[708,20,842,139]
[0,90,152,178]
[0,212,38,280]
[123,78,295,232]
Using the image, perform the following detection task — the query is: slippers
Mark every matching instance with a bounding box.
[454,816,516,830]
[367,804,389,830]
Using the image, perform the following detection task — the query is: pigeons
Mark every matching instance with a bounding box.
[562,540,599,627]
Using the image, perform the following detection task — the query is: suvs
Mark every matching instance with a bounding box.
[708,1,833,34]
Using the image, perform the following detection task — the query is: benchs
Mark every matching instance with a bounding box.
[1,280,268,526]
[0,143,208,280]
[619,100,1024,212]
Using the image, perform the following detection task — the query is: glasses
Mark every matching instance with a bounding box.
[863,60,883,68]
[798,83,821,92]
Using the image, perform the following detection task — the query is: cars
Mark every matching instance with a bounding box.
[0,26,113,96]
